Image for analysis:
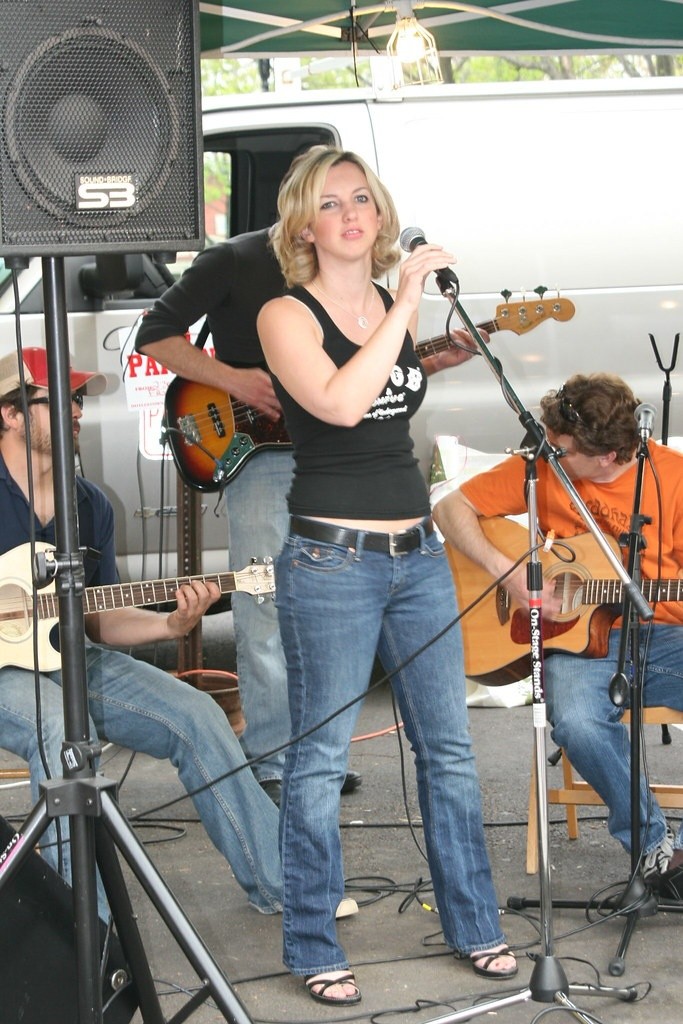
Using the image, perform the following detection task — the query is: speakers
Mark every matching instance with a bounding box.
[0,1,205,264]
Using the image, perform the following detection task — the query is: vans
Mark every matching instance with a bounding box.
[0,76,682,617]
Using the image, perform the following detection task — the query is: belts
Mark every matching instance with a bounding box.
[289,515,433,556]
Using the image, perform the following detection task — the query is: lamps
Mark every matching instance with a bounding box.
[372,3,443,92]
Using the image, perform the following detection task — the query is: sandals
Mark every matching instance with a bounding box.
[304,966,361,1005]
[468,948,518,980]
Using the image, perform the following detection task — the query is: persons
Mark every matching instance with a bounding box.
[0,348,361,927]
[434,373,683,901]
[133,225,361,806]
[256,146,518,1006]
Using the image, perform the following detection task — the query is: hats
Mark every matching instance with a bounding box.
[0,345,107,399]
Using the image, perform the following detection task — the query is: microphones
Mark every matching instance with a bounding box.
[634,403,657,443]
[401,226,460,285]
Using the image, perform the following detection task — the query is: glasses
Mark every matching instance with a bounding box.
[18,390,83,411]
[556,384,586,428]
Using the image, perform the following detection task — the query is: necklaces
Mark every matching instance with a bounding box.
[312,281,376,329]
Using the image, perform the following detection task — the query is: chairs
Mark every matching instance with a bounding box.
[523,700,683,871]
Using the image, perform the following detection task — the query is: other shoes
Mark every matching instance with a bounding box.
[342,770,362,792]
[336,895,359,919]
[259,779,283,806]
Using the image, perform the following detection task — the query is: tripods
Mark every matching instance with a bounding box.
[0,258,254,1024]
[431,278,682,1024]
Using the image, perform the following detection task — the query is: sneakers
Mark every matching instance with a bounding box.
[640,824,676,883]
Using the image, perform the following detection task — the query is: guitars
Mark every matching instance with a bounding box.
[0,540,279,676]
[163,283,576,494]
[443,515,683,689]
